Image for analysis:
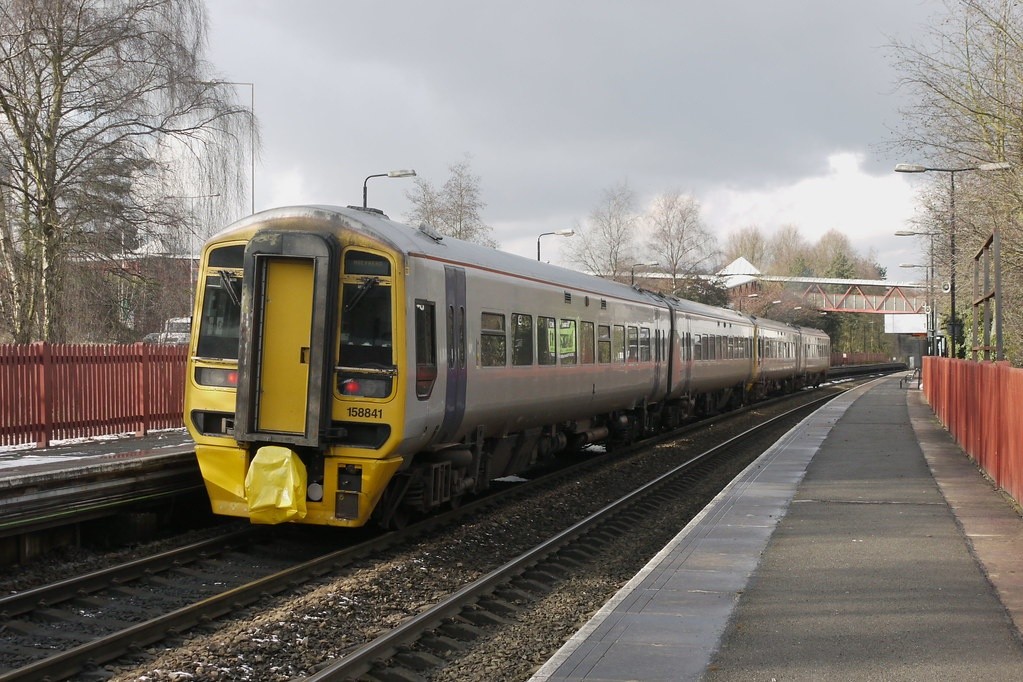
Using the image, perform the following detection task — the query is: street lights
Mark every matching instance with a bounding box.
[895,161,1012,366]
[629,260,661,288]
[361,169,419,216]
[536,230,574,260]
[892,228,949,355]
[164,192,222,318]
[899,263,946,354]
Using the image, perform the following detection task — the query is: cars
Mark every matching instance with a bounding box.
[144,332,160,344]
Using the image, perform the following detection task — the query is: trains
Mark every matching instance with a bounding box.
[181,203,831,529]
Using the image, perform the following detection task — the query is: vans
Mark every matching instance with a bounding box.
[160,316,193,347]
